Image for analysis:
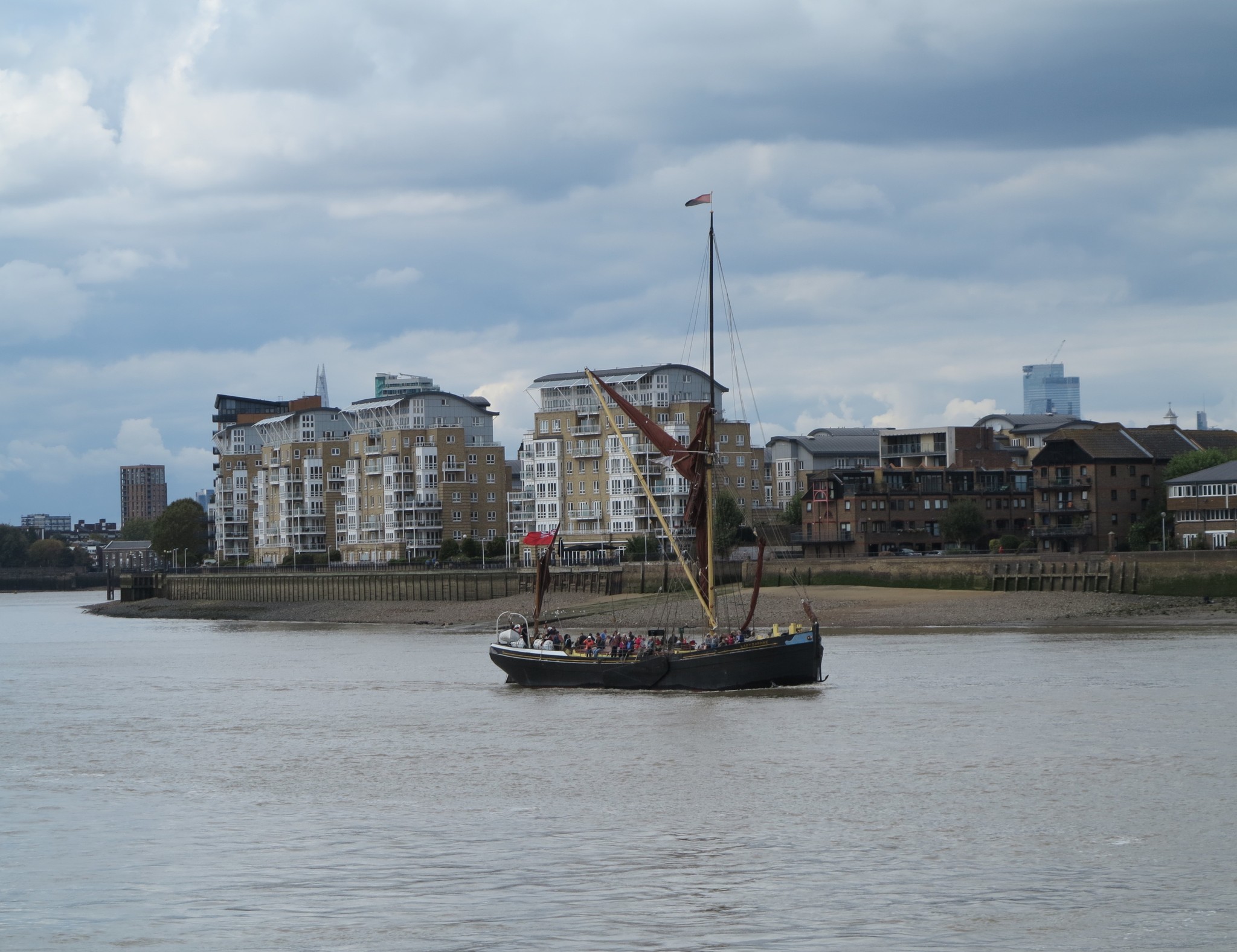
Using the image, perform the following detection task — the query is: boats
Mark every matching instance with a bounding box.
[490,192,829,693]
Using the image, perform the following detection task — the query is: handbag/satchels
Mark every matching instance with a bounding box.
[704,645,707,650]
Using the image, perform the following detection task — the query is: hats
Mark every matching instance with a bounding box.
[588,636,591,639]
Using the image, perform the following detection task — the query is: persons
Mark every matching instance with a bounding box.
[521,623,757,661]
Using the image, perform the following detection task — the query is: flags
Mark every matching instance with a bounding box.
[523,527,557,545]
[685,194,710,206]
